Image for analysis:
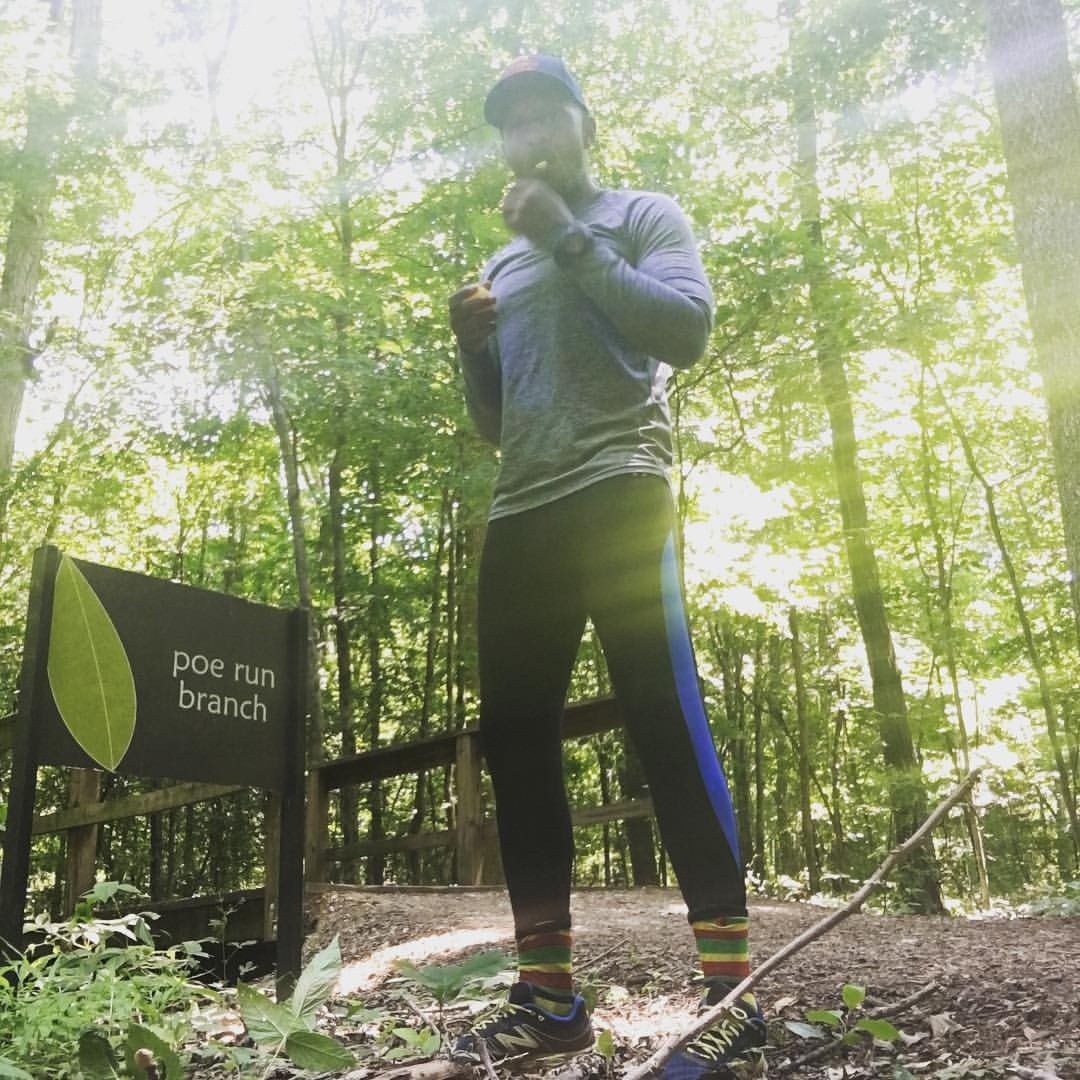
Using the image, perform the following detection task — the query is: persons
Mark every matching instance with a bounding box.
[444,52,767,1080]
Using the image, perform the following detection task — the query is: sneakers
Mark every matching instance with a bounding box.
[658,991,768,1079]
[451,979,597,1070]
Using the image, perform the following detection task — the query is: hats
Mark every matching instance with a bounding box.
[483,55,594,128]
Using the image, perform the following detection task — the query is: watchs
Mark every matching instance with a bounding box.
[552,222,596,270]
